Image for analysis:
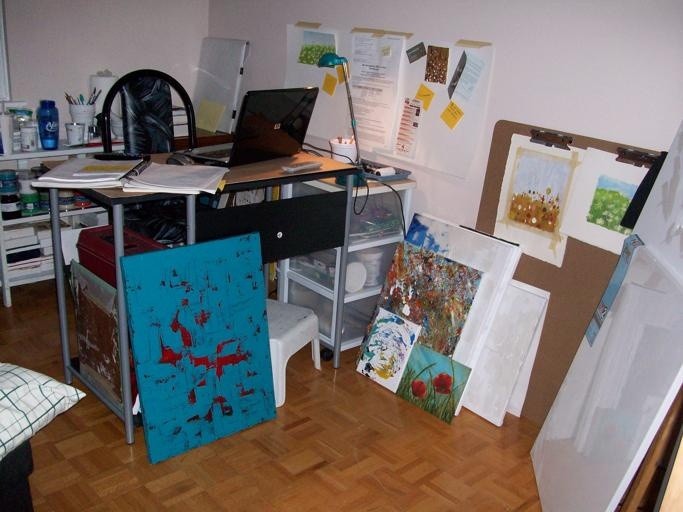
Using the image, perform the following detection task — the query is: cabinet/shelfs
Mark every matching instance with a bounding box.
[0,126,235,309]
[277,179,417,368]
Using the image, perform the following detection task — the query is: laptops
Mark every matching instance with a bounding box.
[184,87,320,167]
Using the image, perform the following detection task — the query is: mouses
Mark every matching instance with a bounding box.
[166,154,193,166]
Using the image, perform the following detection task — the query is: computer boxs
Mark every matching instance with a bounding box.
[76,223,170,291]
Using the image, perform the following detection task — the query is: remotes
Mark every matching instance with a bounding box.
[282,162,323,174]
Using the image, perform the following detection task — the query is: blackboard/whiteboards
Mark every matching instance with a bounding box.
[193,37,247,134]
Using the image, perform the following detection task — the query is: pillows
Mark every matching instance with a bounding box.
[0,361,87,462]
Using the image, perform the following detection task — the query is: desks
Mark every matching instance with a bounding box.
[39,141,363,446]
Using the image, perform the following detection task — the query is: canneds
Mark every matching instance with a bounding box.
[1,185,24,220]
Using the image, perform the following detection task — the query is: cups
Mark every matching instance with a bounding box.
[64,120,86,145]
[329,138,358,165]
[68,104,96,140]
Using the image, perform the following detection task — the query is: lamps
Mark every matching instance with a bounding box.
[317,52,367,187]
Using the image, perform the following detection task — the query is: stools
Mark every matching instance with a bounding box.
[265,298,321,408]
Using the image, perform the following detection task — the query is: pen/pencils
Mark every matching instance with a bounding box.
[64,87,103,104]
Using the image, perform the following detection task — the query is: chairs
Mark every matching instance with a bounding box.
[100,69,197,249]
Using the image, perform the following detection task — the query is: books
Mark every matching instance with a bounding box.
[3,220,87,278]
[30,158,231,196]
[172,106,189,138]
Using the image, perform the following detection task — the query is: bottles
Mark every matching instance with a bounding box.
[0,166,91,220]
[20,127,38,152]
[37,99,59,151]
